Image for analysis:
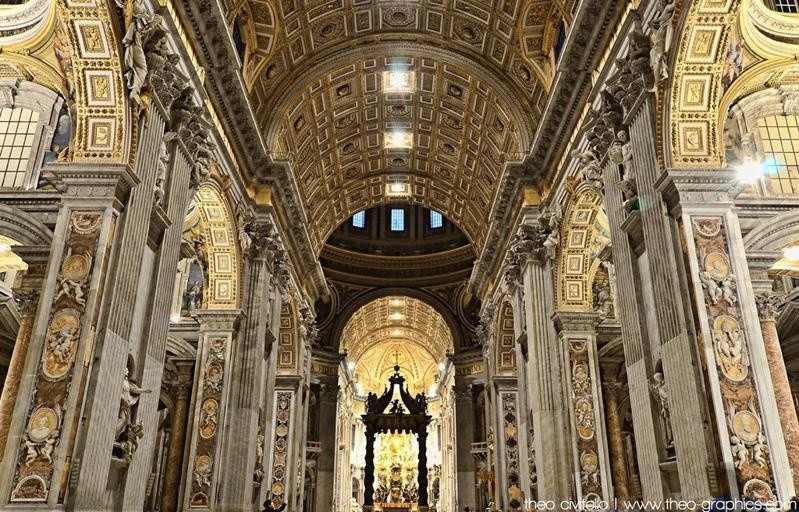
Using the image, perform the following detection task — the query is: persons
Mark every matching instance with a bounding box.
[122,0,217,190]
[23,435,40,467]
[112,366,152,443]
[752,432,770,470]
[571,367,601,487]
[566,0,680,199]
[31,414,51,441]
[652,370,675,450]
[239,208,294,281]
[709,259,726,278]
[60,326,81,359]
[40,429,60,464]
[722,272,737,308]
[124,420,143,458]
[715,331,732,357]
[48,337,67,363]
[727,329,742,356]
[699,270,722,306]
[68,277,87,309]
[594,281,611,314]
[54,275,71,302]
[495,210,559,288]
[729,435,749,472]
[739,414,757,441]
